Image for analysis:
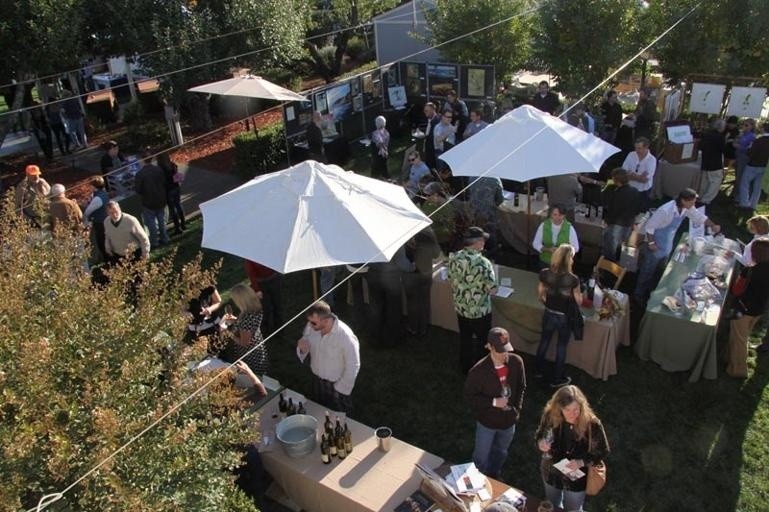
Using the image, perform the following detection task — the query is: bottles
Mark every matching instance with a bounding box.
[336,428,345,459]
[297,402,306,414]
[588,278,595,301]
[581,279,587,298]
[580,291,584,304]
[675,243,689,263]
[342,424,352,453]
[287,397,295,416]
[323,411,334,434]
[278,393,286,412]
[586,199,591,217]
[598,200,603,220]
[335,417,343,434]
[320,433,331,463]
[514,188,519,206]
[327,431,337,456]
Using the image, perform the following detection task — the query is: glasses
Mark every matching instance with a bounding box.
[307,319,323,326]
[409,155,420,162]
[634,163,640,173]
[444,115,453,118]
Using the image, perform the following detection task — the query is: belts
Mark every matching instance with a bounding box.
[187,317,220,332]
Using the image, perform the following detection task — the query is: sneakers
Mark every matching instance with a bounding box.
[530,368,542,380]
[548,377,570,390]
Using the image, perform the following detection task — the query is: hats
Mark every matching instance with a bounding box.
[727,115,739,126]
[486,327,514,353]
[104,140,117,150]
[26,165,42,178]
[423,181,441,195]
[462,226,491,245]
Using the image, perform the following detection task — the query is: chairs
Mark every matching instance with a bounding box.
[595,255,627,290]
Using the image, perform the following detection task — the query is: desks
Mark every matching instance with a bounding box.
[150,331,286,423]
[637,234,740,383]
[427,258,632,379]
[387,460,569,511]
[495,193,658,275]
[296,136,350,166]
[656,147,706,196]
[314,253,447,316]
[83,92,116,122]
[233,389,444,512]
[136,79,160,107]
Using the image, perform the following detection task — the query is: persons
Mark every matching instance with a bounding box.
[464,326,611,512]
[307,82,659,387]
[635,115,769,378]
[185,271,269,399]
[296,301,361,412]
[13,82,184,282]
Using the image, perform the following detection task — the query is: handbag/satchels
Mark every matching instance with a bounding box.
[571,303,584,340]
[585,458,607,497]
[722,307,743,321]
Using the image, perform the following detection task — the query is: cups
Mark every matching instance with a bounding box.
[375,428,392,452]
[536,186,544,201]
[692,237,707,254]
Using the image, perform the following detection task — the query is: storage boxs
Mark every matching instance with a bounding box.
[665,120,700,161]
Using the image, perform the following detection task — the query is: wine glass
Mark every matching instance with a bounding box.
[543,432,554,459]
[537,501,554,512]
[501,384,512,410]
